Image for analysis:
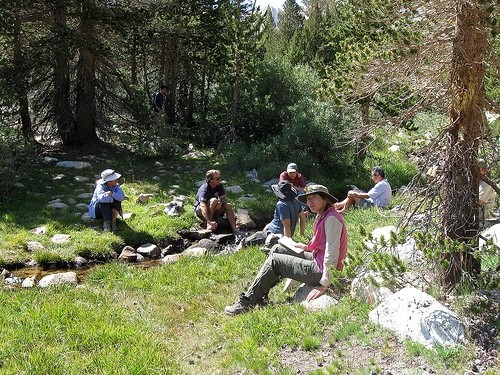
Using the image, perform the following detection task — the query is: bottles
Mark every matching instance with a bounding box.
[252,169,257,180]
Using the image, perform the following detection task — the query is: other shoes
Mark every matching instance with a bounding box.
[225,292,268,316]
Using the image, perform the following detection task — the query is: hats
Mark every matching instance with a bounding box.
[286,163,298,173]
[97,169,121,184]
[297,184,337,206]
[271,180,296,200]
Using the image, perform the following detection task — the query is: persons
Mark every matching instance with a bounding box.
[88,169,125,233]
[193,170,241,232]
[151,85,169,113]
[247,180,305,239]
[332,166,391,213]
[476,158,495,219]
[225,183,348,316]
[280,163,310,216]
